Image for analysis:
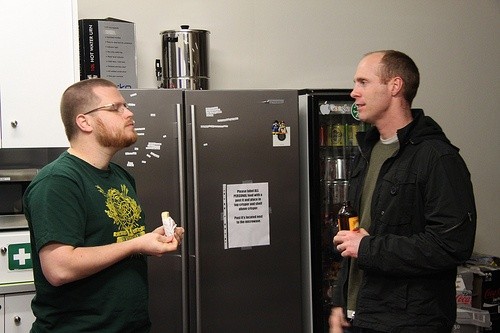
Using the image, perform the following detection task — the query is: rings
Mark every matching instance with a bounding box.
[338,244,342,250]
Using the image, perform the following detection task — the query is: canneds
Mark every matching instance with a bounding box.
[320,156,355,204]
[318,123,361,147]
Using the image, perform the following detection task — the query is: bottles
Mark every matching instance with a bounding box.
[337,200,360,234]
[324,155,349,206]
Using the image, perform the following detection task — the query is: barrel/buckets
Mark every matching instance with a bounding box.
[155,25,209,90]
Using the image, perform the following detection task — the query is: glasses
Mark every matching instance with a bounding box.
[84,99,130,115]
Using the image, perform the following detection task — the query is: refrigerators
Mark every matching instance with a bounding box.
[115,88,304,333]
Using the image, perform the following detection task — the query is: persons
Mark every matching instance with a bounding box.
[23,79,185,333]
[327,49,477,333]
